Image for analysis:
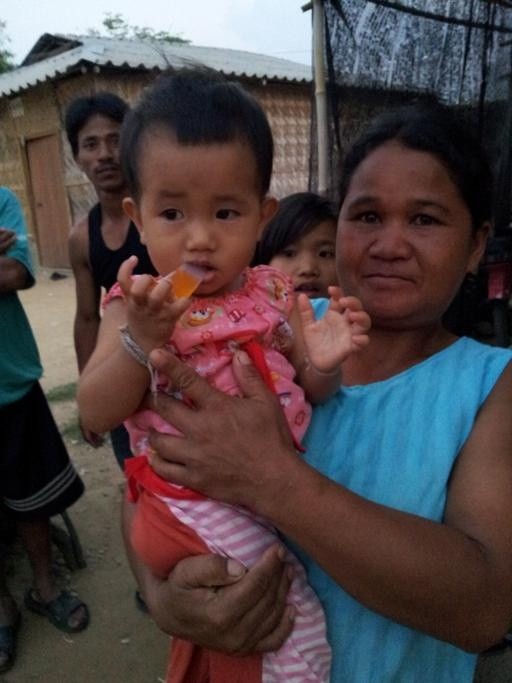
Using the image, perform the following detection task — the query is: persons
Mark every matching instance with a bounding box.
[62,90,150,615]
[253,187,342,304]
[0,184,90,677]
[141,104,512,683]
[74,62,375,683]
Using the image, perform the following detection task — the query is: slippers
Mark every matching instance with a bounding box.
[24,582,90,633]
[0,600,20,674]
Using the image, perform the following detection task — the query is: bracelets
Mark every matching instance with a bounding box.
[304,356,346,376]
[117,322,160,373]
